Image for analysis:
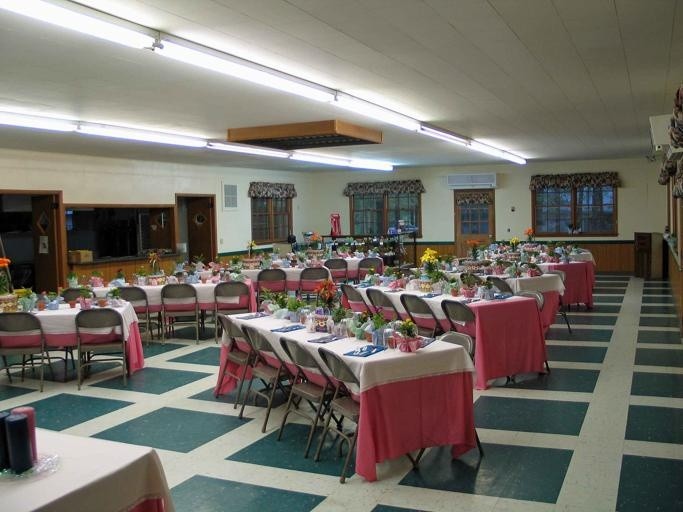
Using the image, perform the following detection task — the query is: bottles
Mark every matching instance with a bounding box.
[4,414,32,471]
[11,406,37,461]
[0,411,10,472]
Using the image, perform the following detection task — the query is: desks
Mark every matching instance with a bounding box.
[0,408,180,512]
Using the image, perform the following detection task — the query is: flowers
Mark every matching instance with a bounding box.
[523,228,535,243]
[310,232,320,241]
[466,240,480,260]
[247,241,257,248]
[510,237,519,252]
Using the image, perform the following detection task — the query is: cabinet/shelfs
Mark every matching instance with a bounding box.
[323,232,417,278]
[634,232,664,281]
